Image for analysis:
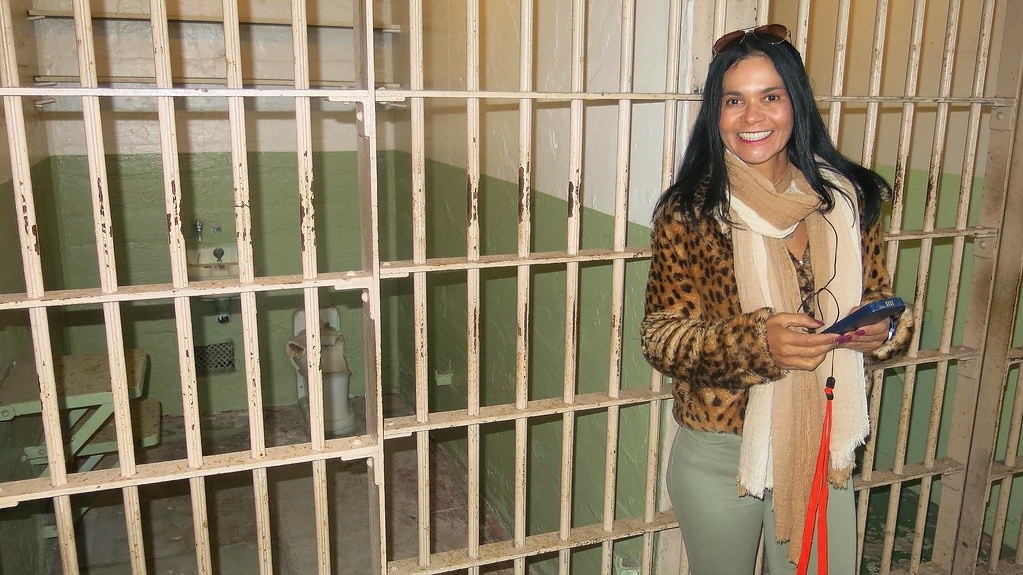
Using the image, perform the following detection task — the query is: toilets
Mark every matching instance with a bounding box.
[285,304,355,435]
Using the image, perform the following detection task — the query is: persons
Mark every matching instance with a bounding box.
[638,31,914,575]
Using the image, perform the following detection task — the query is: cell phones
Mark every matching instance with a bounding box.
[820,297,906,336]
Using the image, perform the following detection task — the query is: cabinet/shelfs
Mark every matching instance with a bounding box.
[0,349,160,545]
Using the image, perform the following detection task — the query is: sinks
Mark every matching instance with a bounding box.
[184,262,240,297]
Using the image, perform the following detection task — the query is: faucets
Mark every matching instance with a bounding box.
[191,220,203,242]
